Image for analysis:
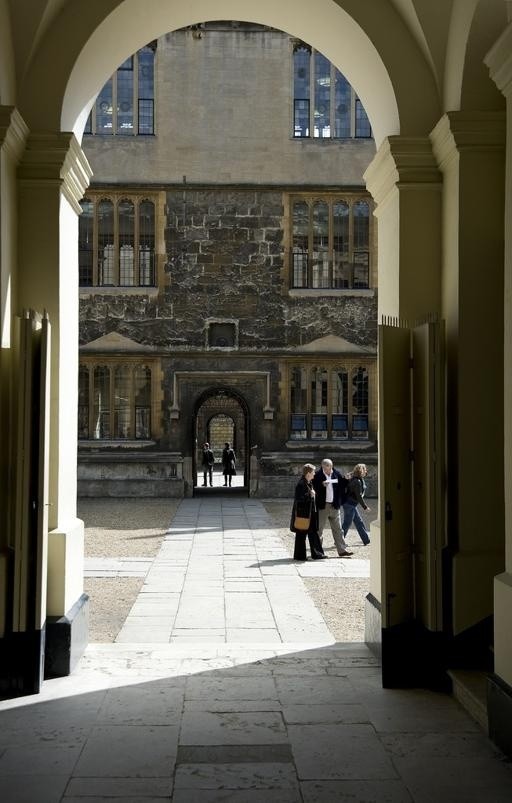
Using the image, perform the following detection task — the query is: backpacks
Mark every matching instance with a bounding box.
[341,475,364,505]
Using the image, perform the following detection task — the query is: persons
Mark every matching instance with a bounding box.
[290,463,328,560]
[310,458,354,557]
[221,441,236,486]
[201,442,214,486]
[333,463,372,547]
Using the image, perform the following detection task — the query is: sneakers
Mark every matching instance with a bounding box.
[202,484,206,486]
[223,483,231,487]
[311,554,329,559]
[339,550,353,556]
[294,556,309,561]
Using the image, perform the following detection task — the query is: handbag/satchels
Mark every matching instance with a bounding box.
[294,517,310,531]
[208,462,213,470]
